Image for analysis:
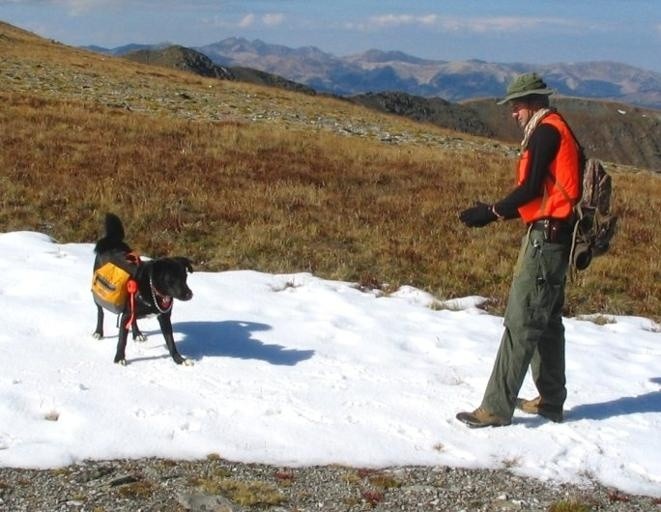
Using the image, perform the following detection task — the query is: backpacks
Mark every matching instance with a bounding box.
[532,110,615,286]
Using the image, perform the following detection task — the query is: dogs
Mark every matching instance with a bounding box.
[92,212,194,367]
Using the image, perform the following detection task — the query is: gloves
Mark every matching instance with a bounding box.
[459,201,498,228]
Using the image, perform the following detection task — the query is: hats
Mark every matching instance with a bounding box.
[496,73,553,106]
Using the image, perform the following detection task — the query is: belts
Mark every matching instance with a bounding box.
[529,222,572,232]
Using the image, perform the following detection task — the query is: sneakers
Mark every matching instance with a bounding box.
[455,406,511,427]
[514,396,563,423]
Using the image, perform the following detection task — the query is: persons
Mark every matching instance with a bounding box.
[456,72,583,428]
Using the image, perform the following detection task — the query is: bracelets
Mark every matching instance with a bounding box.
[492,205,501,217]
